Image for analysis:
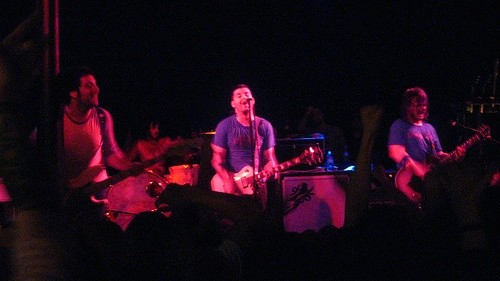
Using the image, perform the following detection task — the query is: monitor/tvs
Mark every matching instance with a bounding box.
[280,171,349,234]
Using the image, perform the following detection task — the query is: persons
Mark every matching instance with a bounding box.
[210,83,278,232]
[299,105,346,171]
[2,10,61,199]
[128,114,185,170]
[386,87,459,214]
[0,104,500,281]
[32,65,163,217]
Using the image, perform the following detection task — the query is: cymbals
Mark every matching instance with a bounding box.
[202,131,216,134]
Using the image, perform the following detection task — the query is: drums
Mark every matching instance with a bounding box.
[102,169,173,226]
[169,164,201,185]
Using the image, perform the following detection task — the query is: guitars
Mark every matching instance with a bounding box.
[431,124,492,169]
[211,144,324,196]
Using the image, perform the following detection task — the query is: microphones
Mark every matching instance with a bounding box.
[241,97,253,105]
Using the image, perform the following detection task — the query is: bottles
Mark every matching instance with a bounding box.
[325,151,334,171]
